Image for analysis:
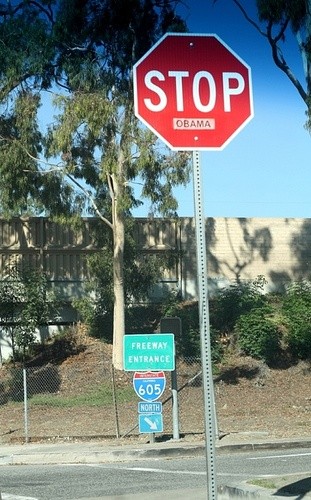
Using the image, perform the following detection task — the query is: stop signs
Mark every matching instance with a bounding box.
[133,33,254,151]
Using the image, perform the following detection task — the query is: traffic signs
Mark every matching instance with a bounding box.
[123,334,175,371]
[133,371,166,402]
[138,401,162,414]
[138,414,163,434]
